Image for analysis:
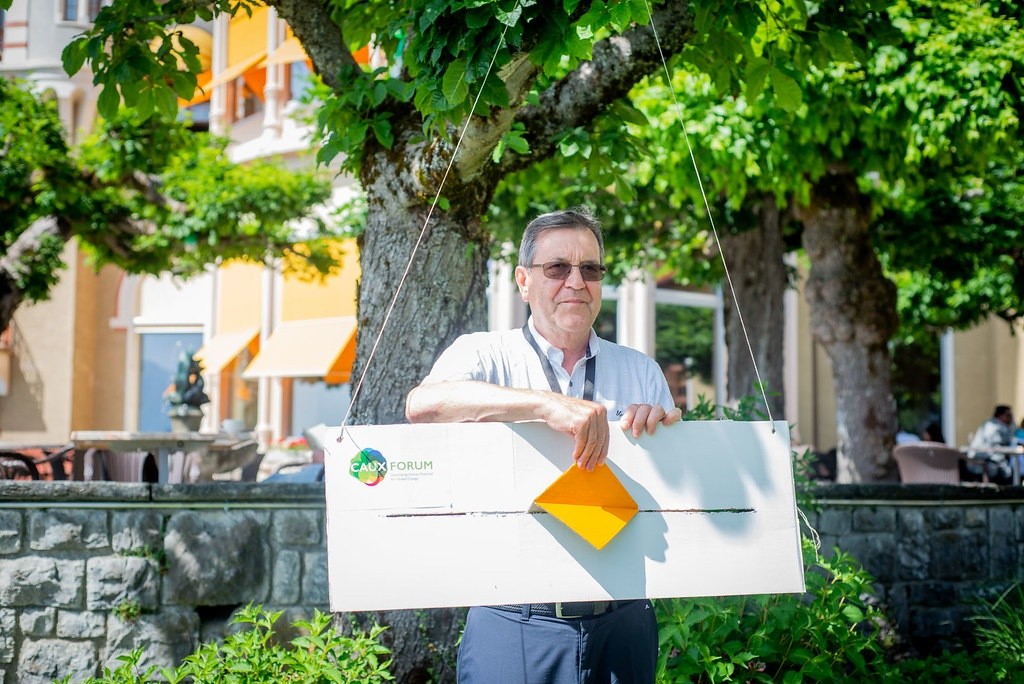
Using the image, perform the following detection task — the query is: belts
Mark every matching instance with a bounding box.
[481,600,640,619]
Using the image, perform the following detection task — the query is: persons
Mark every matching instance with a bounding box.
[406,211,684,684]
[968,404,1024,486]
[922,421,945,444]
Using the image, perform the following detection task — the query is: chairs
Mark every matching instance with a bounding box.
[891,442,1000,491]
[0,429,325,483]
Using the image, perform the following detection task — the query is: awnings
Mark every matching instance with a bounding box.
[241,316,358,383]
[258,36,370,62]
[195,324,260,376]
[180,51,267,107]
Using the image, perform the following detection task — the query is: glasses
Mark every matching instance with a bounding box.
[523,261,608,281]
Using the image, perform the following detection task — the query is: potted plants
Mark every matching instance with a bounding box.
[165,352,210,433]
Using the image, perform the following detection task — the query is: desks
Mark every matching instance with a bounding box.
[71,428,216,485]
[958,444,1024,483]
